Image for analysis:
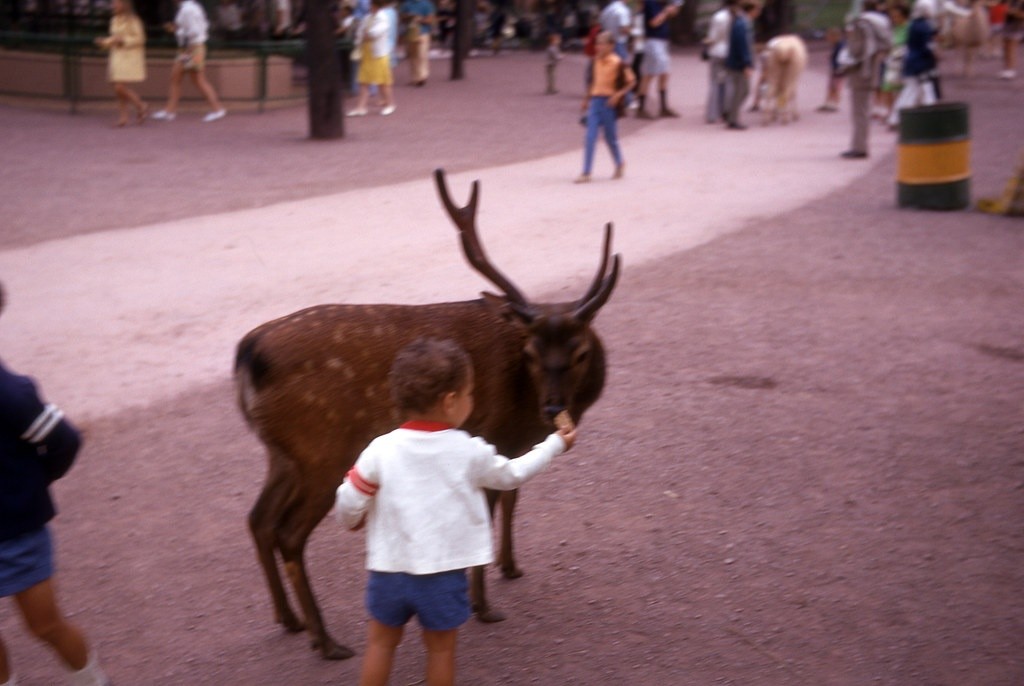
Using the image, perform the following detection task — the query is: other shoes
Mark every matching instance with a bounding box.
[841,150,868,158]
[635,111,652,119]
[113,118,130,126]
[661,109,678,117]
[574,175,591,183]
[728,122,747,129]
[612,172,622,178]
[136,103,155,124]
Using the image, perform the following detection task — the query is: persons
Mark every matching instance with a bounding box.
[96,1,150,128]
[579,31,637,184]
[544,33,564,94]
[151,1,227,122]
[579,0,1024,159]
[0,282,103,686]
[216,0,436,116]
[333,338,579,686]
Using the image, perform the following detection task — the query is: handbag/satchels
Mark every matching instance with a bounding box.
[173,53,200,82]
[926,74,942,99]
[350,45,363,60]
[399,16,422,44]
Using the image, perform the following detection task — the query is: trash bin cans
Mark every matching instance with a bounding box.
[896,101,971,213]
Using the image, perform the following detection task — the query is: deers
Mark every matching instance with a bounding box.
[232,167,624,665]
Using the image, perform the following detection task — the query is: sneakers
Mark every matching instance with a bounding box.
[346,107,369,116]
[203,108,226,121]
[151,109,176,121]
[380,105,397,115]
